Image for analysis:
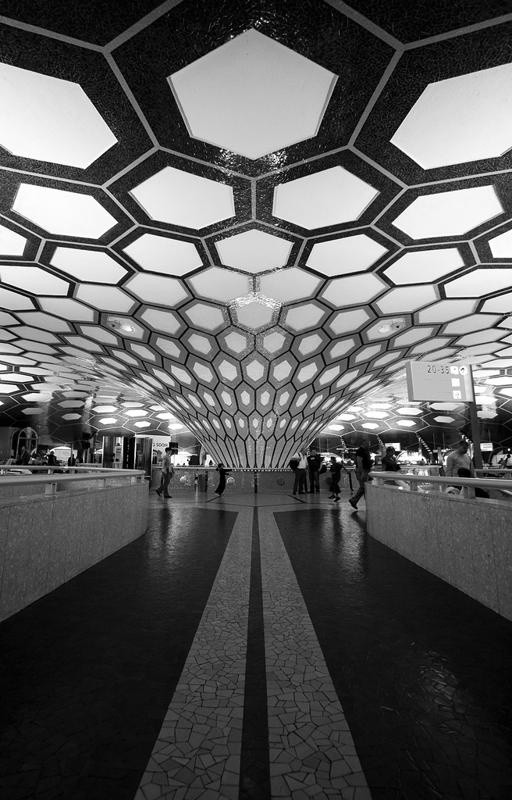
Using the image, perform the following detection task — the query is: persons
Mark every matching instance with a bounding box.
[288,436,512,509]
[11,447,117,474]
[151,446,234,497]
